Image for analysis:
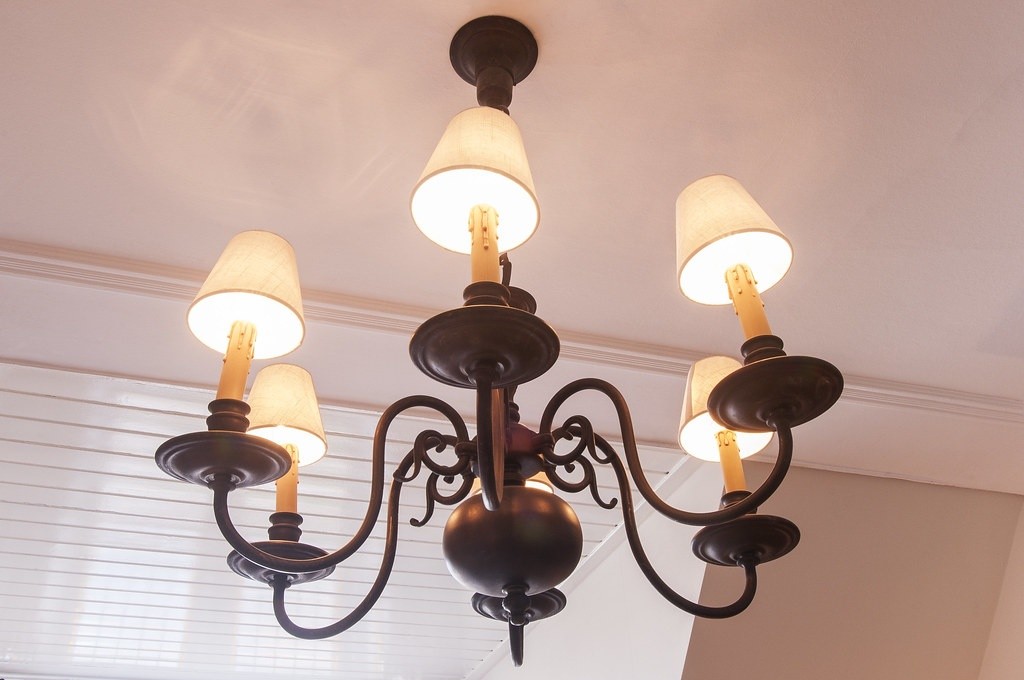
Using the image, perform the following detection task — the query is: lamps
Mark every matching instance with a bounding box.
[152,13,846,668]
